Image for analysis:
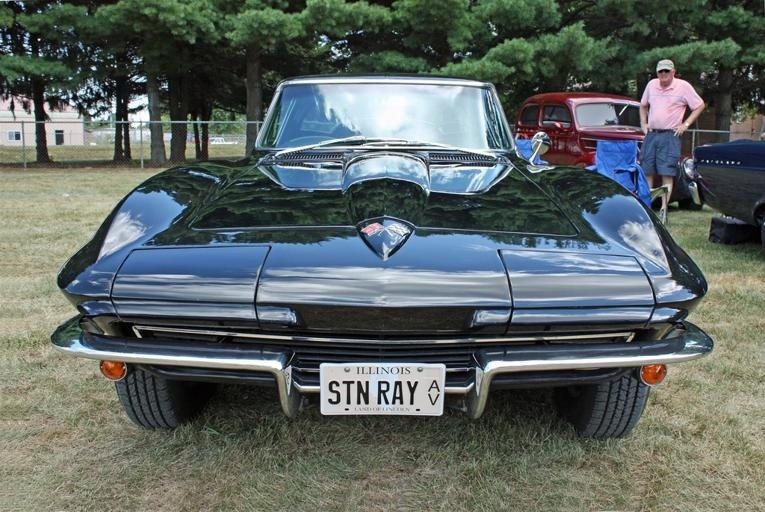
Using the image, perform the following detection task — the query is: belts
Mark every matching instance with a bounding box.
[649,129,672,133]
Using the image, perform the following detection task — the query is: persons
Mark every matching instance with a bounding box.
[634,57,707,215]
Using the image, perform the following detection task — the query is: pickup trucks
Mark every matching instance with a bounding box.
[209,137,238,145]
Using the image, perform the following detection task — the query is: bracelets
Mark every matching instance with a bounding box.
[684,121,692,127]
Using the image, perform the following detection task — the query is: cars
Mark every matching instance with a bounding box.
[695,139,765,243]
[512,93,705,210]
[49,78,716,444]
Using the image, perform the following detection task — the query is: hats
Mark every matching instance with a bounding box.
[655,59,675,73]
